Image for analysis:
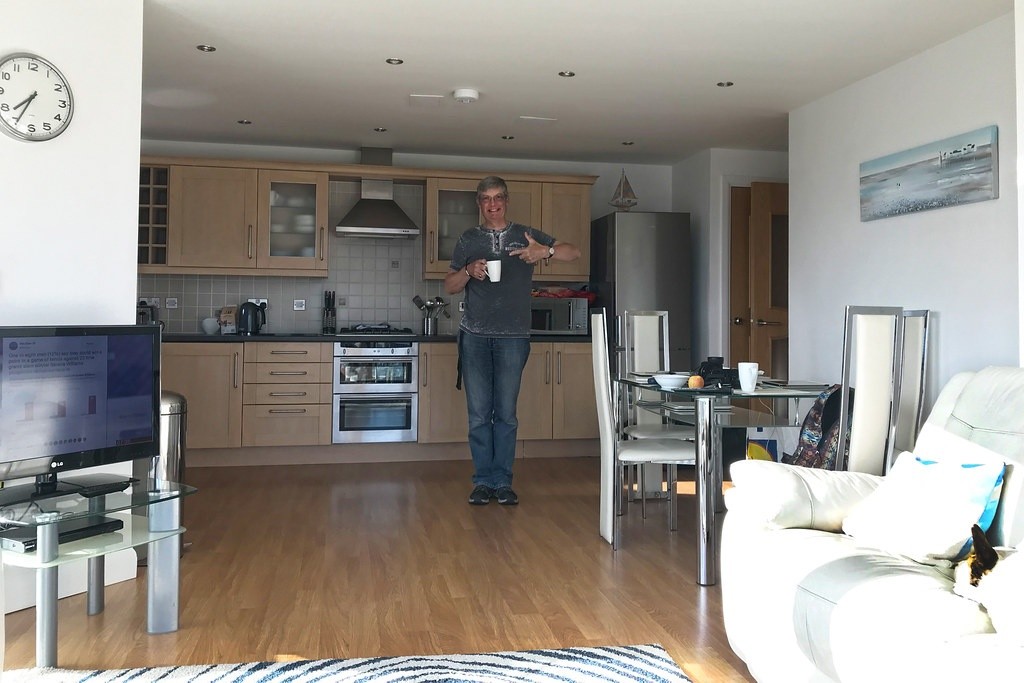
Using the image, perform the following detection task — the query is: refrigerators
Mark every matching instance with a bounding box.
[590,211,693,374]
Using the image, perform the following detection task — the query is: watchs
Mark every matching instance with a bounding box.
[547,246,555,258]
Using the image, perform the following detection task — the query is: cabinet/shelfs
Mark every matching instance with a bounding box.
[421,178,482,279]
[418,343,471,444]
[514,342,602,441]
[505,180,591,282]
[257,169,332,278]
[164,164,258,276]
[161,343,244,450]
[241,342,331,447]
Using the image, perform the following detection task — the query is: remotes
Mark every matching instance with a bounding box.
[78,480,131,499]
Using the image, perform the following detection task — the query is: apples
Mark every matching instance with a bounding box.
[688,375,704,388]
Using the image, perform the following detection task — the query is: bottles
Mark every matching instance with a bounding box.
[302,246,315,257]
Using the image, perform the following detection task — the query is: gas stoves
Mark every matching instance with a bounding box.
[334,327,417,337]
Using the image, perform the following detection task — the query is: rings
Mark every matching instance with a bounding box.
[479,274,482,278]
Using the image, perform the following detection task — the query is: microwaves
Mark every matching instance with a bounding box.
[530,295,588,335]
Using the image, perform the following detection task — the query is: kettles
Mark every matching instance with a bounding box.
[238,301,266,336]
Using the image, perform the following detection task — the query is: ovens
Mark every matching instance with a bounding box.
[333,342,418,443]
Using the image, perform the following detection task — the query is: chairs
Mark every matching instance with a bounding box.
[587,303,931,551]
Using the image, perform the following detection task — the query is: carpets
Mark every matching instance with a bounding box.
[0,645,688,683]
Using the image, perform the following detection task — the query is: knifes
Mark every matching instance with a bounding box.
[325,290,336,311]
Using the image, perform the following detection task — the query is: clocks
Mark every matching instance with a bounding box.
[0,52,74,142]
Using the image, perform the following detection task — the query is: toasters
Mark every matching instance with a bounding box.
[136,305,159,325]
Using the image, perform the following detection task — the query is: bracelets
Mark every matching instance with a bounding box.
[465,263,472,278]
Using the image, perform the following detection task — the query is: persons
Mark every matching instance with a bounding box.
[444,176,581,505]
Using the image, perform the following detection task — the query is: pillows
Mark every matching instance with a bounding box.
[840,450,1006,563]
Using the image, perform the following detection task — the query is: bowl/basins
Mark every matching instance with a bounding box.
[271,214,315,233]
[287,199,305,208]
[652,375,690,389]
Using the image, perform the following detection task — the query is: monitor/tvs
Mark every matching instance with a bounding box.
[0,322,162,504]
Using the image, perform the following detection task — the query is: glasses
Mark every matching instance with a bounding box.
[481,193,503,203]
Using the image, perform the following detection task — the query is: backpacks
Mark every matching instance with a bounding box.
[787,384,855,471]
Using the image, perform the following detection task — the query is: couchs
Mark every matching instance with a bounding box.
[720,362,1024,683]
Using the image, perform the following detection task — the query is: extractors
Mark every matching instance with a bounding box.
[335,147,421,240]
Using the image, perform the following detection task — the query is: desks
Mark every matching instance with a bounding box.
[0,473,191,669]
[612,372,835,586]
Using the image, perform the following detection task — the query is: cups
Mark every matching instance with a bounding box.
[738,362,759,394]
[201,318,220,335]
[271,190,279,206]
[483,260,501,282]
[423,317,438,335]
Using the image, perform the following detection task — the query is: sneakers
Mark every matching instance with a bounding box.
[493,486,519,505]
[468,485,494,504]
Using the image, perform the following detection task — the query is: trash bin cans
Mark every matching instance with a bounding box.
[134,390,188,562]
